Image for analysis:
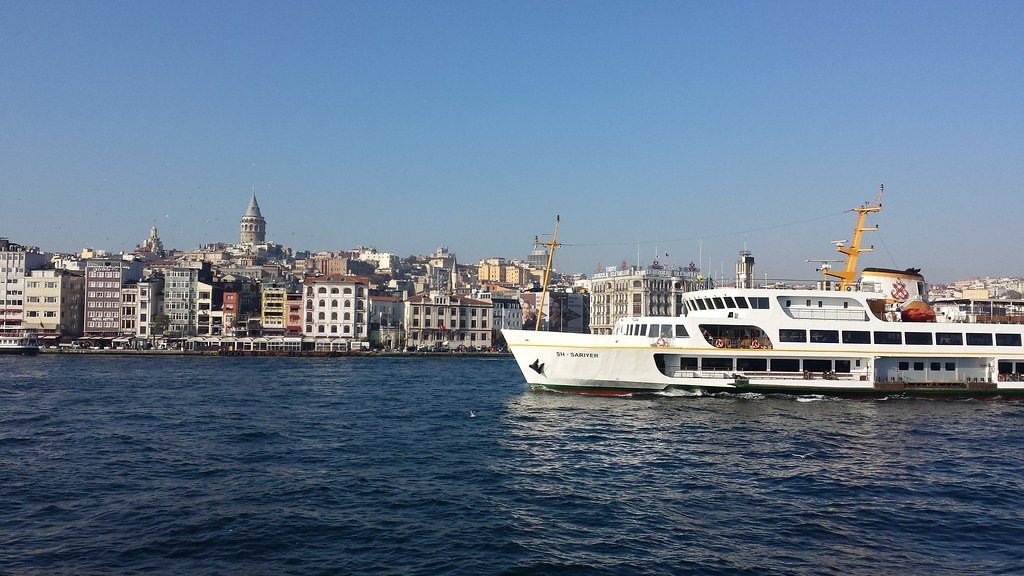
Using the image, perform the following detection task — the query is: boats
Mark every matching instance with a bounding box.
[901,299,935,323]
[0,335,41,356]
[502,182,1024,402]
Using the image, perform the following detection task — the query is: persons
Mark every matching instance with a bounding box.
[458,345,508,353]
[550,300,567,330]
[522,301,535,330]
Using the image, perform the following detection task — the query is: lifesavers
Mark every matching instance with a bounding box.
[715,339,723,348]
[751,340,761,349]
[657,338,665,346]
[999,374,1005,381]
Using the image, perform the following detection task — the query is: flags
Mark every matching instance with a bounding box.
[440,325,446,331]
